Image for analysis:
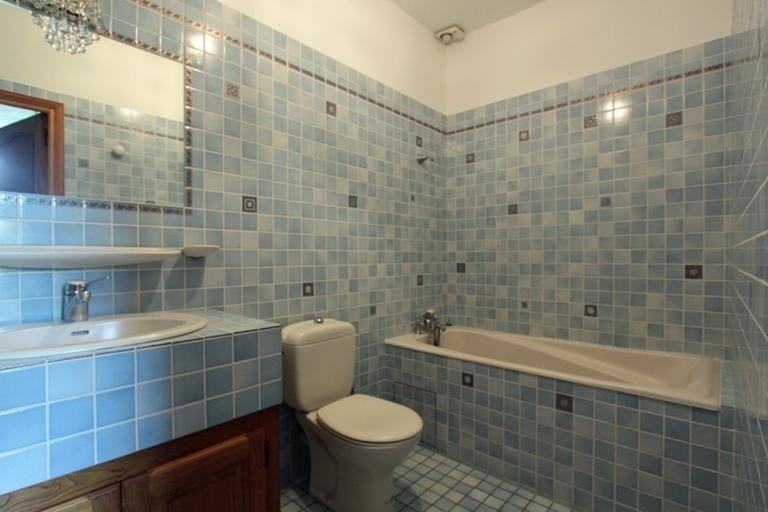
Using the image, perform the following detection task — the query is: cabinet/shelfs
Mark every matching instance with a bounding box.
[0,406,282,512]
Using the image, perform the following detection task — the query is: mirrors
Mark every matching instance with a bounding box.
[0,0,198,214]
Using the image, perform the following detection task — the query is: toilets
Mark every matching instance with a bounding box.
[280,318,424,512]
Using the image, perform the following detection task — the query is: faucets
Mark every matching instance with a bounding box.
[62,275,112,321]
[414,309,449,343]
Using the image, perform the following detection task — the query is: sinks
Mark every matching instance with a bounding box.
[0,312,207,361]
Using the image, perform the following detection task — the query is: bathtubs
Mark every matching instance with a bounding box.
[384,325,723,416]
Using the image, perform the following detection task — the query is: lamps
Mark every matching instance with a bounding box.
[28,0,102,53]
[435,25,465,44]
[31,11,100,54]
